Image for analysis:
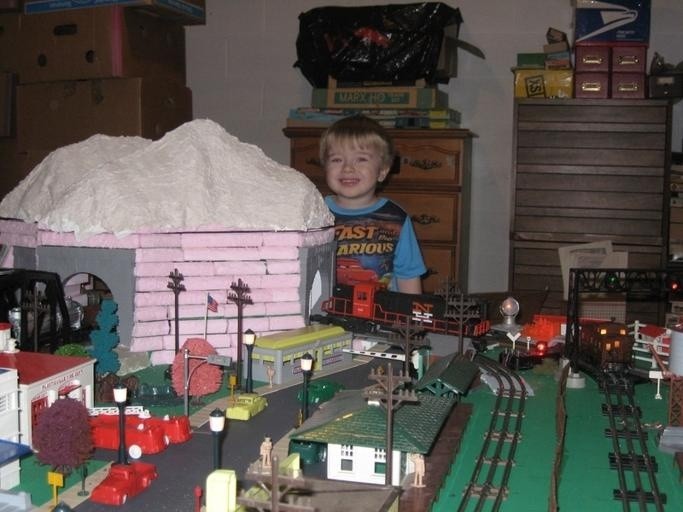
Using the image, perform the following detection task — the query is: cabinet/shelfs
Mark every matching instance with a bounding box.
[507,96,673,324]
[281,128,478,296]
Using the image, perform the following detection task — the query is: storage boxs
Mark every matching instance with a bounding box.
[284,2,464,129]
[511,0,653,99]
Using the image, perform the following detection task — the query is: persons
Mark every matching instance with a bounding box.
[319,119,427,296]
